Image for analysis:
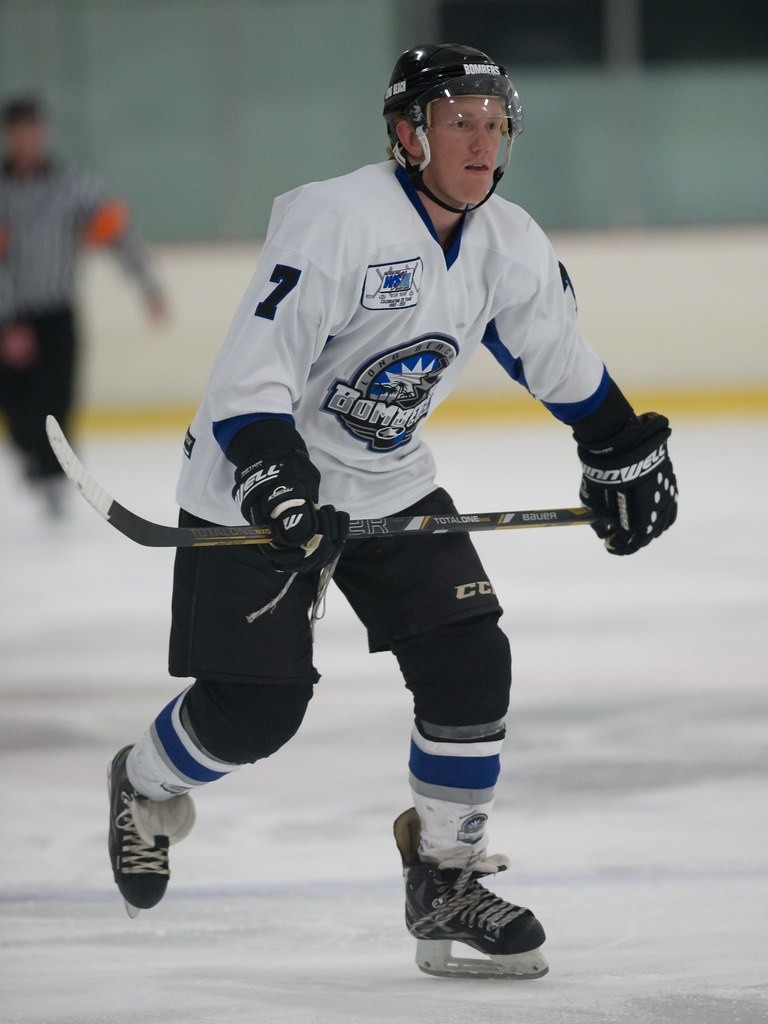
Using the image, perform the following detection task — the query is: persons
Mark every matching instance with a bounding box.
[110,41,678,979]
[0,95,168,509]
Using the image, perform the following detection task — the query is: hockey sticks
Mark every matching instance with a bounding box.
[43,414,593,548]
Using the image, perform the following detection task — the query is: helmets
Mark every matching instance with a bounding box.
[383,43,520,139]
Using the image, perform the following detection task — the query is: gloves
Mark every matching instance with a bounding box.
[578,412,679,555]
[231,448,348,576]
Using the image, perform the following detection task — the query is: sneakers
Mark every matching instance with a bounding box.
[108,744,196,919]
[393,808,549,980]
